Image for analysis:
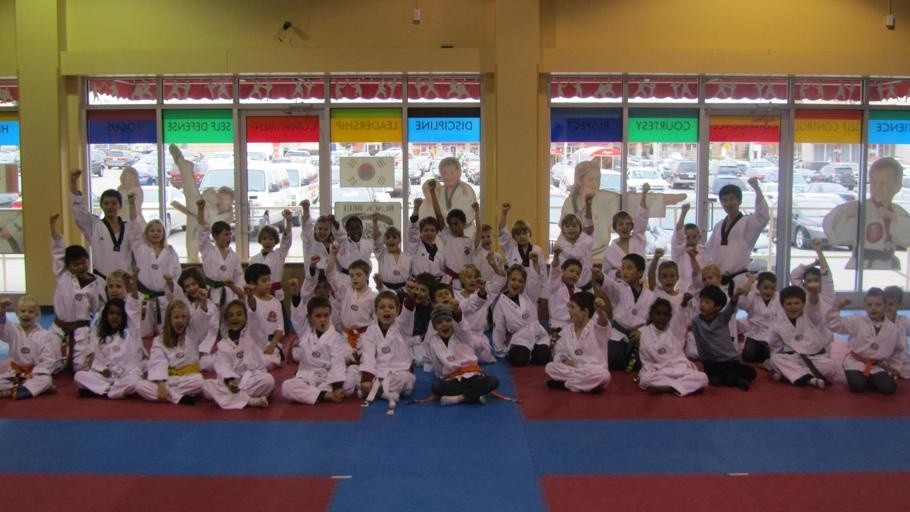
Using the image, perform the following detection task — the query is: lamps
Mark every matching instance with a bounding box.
[273,20,292,44]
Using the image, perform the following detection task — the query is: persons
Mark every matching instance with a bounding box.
[168,143,237,260]
[427,156,478,238]
[1,168,910,410]
[559,151,685,261]
[821,156,910,275]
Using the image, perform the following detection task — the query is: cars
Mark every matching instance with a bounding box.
[554,147,742,198]
[743,149,910,252]
[353,145,497,199]
[89,140,203,234]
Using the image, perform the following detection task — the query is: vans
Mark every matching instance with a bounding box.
[203,142,345,239]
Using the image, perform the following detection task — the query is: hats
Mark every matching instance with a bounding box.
[431,303,455,330]
